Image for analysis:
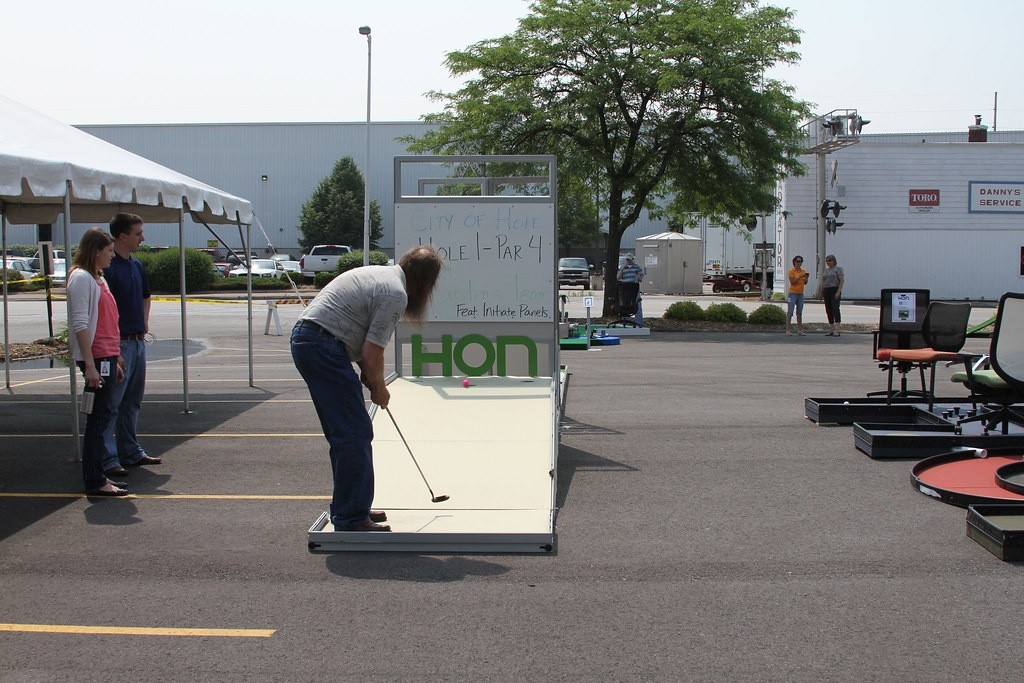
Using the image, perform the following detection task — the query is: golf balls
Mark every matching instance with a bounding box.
[462,380,469,387]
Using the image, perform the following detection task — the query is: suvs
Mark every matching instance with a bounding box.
[558,257,594,289]
[603,255,641,280]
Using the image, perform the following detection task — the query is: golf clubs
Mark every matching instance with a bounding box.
[385,405,451,503]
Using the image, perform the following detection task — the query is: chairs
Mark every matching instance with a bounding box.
[947,291,1024,435]
[605,279,645,329]
[890,296,974,408]
[863,286,934,399]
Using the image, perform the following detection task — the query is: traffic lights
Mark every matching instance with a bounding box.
[822,119,838,131]
[861,117,872,133]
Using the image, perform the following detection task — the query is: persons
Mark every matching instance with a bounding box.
[67,227,129,496]
[616,252,644,327]
[101,211,162,477]
[786,256,809,336]
[102,366,108,373]
[289,246,441,531]
[818,255,844,337]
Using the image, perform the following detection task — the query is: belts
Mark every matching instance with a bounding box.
[121,334,144,341]
[295,320,330,334]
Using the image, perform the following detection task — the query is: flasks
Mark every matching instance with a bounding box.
[80,381,96,414]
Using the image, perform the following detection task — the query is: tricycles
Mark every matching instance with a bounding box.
[709,273,753,291]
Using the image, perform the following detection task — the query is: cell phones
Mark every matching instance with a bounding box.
[805,273,809,276]
[624,266,629,269]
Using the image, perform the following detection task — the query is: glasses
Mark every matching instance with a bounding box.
[826,260,832,262]
[795,260,803,262]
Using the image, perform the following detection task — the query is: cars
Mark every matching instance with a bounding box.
[279,260,300,274]
[0,250,66,287]
[214,262,234,278]
[751,270,773,290]
[197,248,260,263]
[270,254,291,261]
[228,259,289,279]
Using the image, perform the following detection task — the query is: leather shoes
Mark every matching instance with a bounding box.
[331,510,386,522]
[334,517,390,531]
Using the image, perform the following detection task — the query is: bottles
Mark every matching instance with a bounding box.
[559,365,566,383]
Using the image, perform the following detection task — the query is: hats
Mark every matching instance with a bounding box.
[625,253,633,260]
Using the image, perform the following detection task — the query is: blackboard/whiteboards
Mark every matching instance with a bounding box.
[393,202,555,323]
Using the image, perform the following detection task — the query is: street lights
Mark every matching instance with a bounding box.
[357,23,373,267]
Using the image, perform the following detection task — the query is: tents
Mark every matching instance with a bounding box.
[0,93,257,463]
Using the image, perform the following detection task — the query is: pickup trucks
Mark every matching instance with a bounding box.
[299,244,355,277]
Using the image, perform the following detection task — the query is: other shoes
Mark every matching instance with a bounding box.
[786,332,792,336]
[833,333,839,337]
[109,466,128,475]
[139,456,161,464]
[798,333,807,336]
[97,480,127,495]
[825,332,834,336]
